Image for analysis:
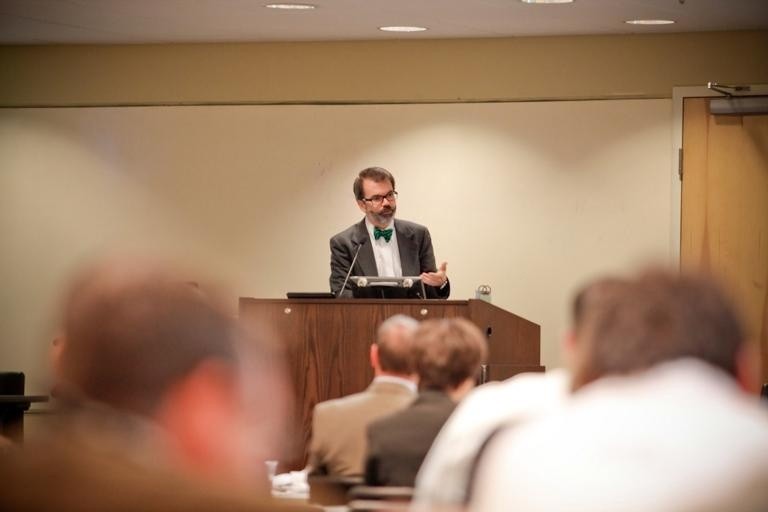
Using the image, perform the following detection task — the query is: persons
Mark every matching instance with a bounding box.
[1,267,325,512]
[411,271,768,512]
[329,167,451,298]
[363,317,486,488]
[304,313,420,485]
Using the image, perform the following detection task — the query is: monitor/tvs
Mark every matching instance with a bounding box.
[350,276,426,300]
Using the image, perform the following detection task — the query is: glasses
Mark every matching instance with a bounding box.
[361,191,398,206]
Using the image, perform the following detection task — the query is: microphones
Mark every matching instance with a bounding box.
[339,235,368,298]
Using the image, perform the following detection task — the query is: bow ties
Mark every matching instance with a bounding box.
[374,226,393,242]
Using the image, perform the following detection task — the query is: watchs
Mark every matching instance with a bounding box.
[440,281,448,290]
